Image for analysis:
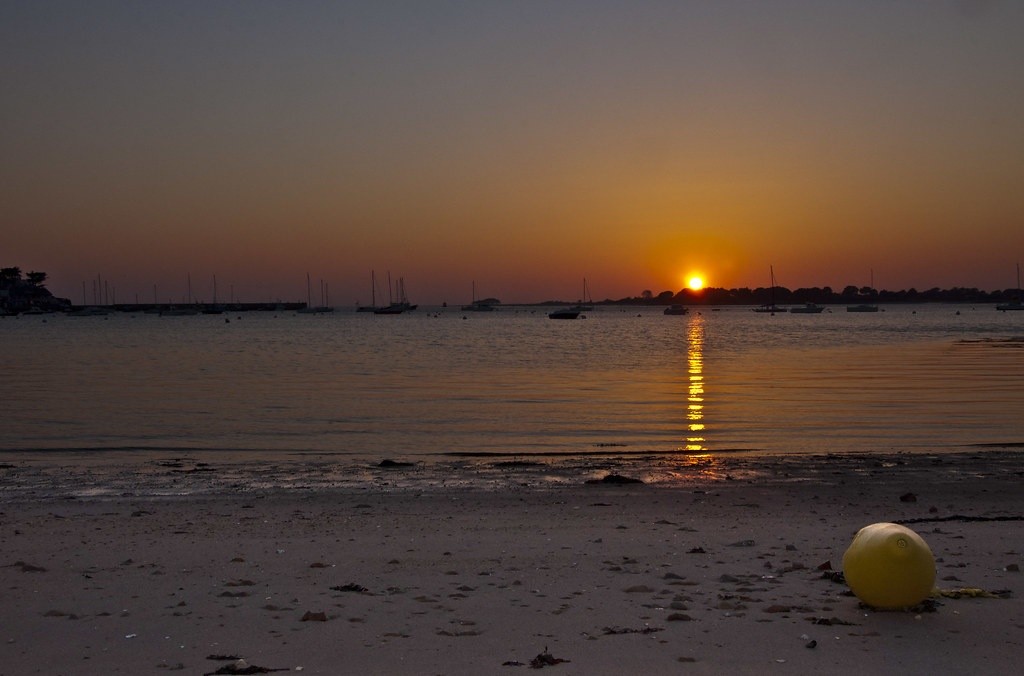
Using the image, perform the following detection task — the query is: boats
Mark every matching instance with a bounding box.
[846,305,878,312]
[790,303,825,313]
[549,311,580,319]
[664,304,689,315]
[752,303,788,312]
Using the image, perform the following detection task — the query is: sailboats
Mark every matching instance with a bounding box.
[569,278,595,311]
[69,272,252,316]
[461,280,493,311]
[996,263,1024,310]
[372,270,418,314]
[298,272,334,313]
